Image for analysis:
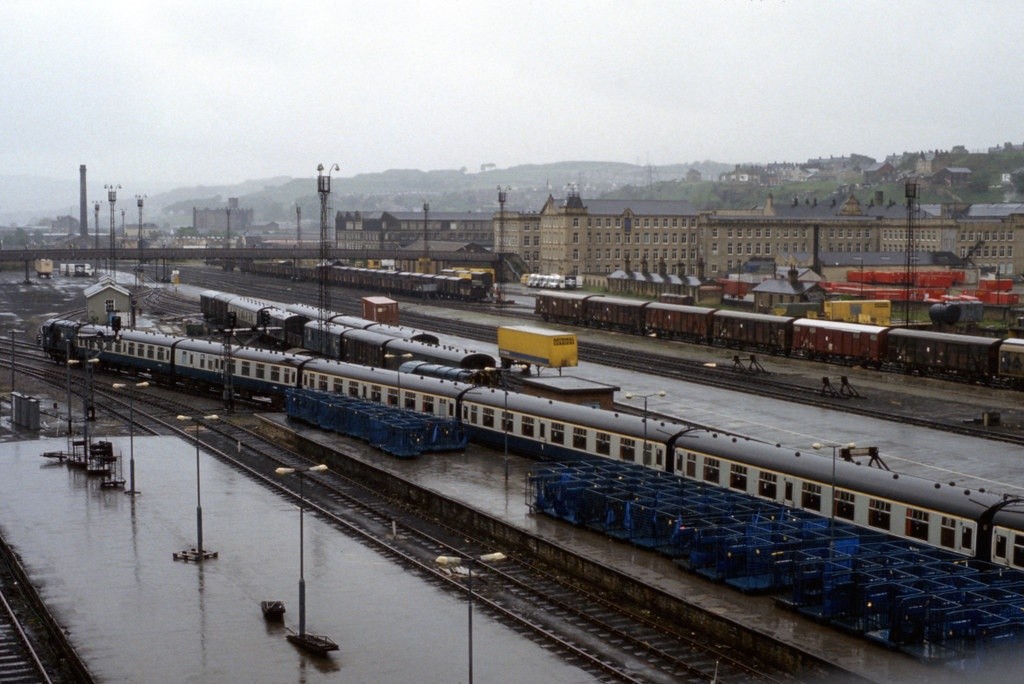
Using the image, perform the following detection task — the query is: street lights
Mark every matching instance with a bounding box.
[295,201,305,255]
[68,358,100,465]
[899,172,922,328]
[436,552,509,684]
[316,162,340,358]
[226,206,231,256]
[104,183,122,279]
[7,328,26,391]
[420,197,434,274]
[813,442,857,561]
[91,200,102,274]
[493,185,513,303]
[134,193,147,286]
[176,415,221,552]
[275,464,329,635]
[113,381,149,493]
[118,208,128,259]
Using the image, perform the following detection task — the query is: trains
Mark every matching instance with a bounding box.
[534,289,1024,390]
[205,256,487,304]
[200,289,560,384]
[36,320,1023,572]
